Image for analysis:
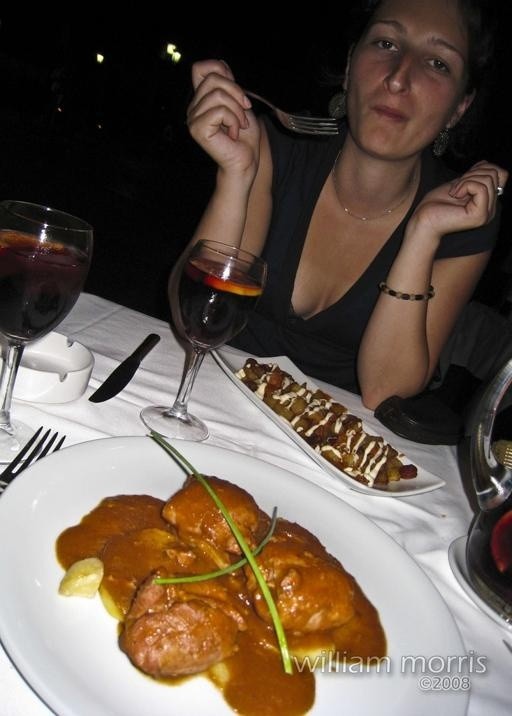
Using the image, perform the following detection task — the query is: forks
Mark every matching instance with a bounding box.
[240,88,341,137]
[0,424,68,496]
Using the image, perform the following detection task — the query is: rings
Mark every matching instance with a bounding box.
[496,187,504,196]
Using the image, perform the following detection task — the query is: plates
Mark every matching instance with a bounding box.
[208,346,447,499]
[0,433,472,715]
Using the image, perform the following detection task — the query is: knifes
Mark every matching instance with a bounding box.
[85,332,163,404]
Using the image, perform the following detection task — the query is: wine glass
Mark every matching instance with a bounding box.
[138,235,268,443]
[0,195,96,465]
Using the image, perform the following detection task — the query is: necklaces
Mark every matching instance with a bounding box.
[330,147,417,222]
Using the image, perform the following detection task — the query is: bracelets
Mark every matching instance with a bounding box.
[376,281,435,303]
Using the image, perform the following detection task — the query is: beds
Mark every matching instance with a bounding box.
[0,291,512,716]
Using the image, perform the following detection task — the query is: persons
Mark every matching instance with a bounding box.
[166,2,507,418]
